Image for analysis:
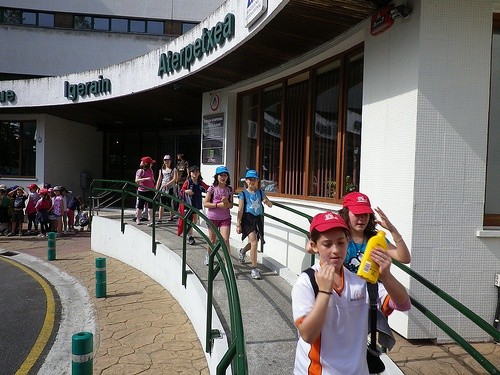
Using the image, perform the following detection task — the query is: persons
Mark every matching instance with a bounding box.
[35,188,54,238]
[78,203,90,231]
[61,187,78,231]
[291,191,411,282]
[180,166,211,244]
[202,166,234,264]
[155,155,177,224]
[44,183,68,232]
[0,184,28,237]
[236,170,273,279]
[24,184,40,234]
[52,186,64,235]
[290,208,411,375]
[174,154,189,190]
[132,157,156,226]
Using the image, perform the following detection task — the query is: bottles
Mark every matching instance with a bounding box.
[356,229,388,284]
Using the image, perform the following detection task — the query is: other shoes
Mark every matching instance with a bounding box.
[239,248,246,264]
[187,235,196,245]
[252,268,263,280]
[205,255,209,265]
[167,218,174,223]
[156,219,162,224]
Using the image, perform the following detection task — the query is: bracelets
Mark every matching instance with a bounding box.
[394,237,402,242]
[216,202,217,208]
[319,290,332,294]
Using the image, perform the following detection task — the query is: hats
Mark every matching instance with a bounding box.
[189,166,200,173]
[37,188,47,195]
[310,212,349,239]
[26,184,37,189]
[141,157,156,164]
[216,166,228,174]
[164,155,172,161]
[343,192,375,215]
[246,170,259,179]
[0,185,7,191]
[53,185,62,191]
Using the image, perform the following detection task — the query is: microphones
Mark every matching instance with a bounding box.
[192,176,195,179]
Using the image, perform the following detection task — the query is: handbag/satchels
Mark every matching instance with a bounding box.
[178,204,185,236]
